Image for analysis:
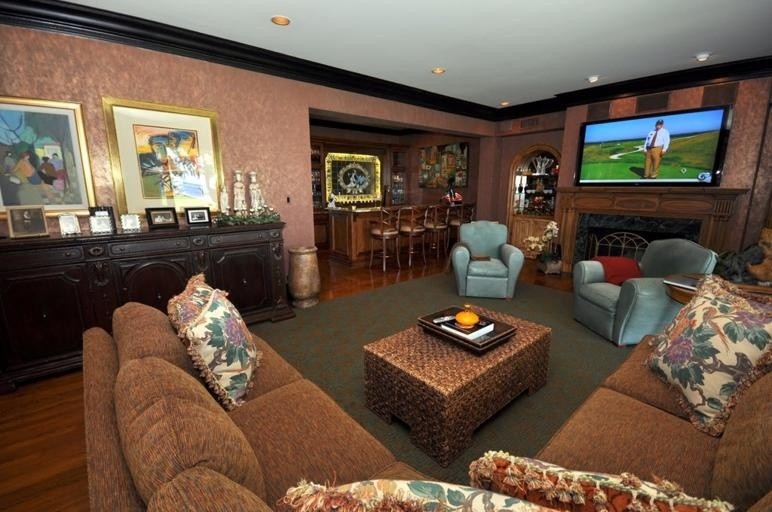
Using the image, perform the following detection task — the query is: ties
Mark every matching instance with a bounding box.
[650,131,658,148]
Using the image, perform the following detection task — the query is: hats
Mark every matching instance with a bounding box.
[656,119,663,124]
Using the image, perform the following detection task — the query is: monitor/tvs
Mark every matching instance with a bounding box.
[574,103,735,186]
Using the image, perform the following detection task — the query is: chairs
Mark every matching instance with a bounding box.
[570,237,721,349]
[368,203,474,271]
[450,219,523,302]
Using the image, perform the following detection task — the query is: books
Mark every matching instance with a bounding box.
[441,317,495,341]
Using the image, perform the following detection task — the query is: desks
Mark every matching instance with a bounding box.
[329,202,469,272]
[662,281,771,311]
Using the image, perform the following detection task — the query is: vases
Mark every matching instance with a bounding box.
[286,245,324,310]
[534,255,563,275]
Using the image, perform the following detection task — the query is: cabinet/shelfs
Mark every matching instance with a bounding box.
[1,219,299,393]
[310,142,326,211]
[512,170,555,212]
[391,146,408,206]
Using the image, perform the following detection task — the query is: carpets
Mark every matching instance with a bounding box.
[248,271,637,485]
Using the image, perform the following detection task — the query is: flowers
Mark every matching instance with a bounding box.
[522,219,564,264]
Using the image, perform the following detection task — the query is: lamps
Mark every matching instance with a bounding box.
[587,74,599,85]
[695,53,711,63]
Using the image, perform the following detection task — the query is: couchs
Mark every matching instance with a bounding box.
[74,300,459,512]
[530,332,772,512]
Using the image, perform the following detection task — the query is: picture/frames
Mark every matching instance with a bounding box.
[101,95,228,222]
[4,205,49,237]
[184,207,212,225]
[144,207,181,231]
[56,204,142,235]
[0,93,98,221]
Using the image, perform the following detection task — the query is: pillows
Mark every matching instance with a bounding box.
[270,464,560,512]
[645,272,772,351]
[168,271,215,336]
[468,449,733,511]
[650,289,771,439]
[180,285,266,414]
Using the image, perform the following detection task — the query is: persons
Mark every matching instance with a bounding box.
[4,151,15,173]
[643,120,670,179]
[49,153,63,170]
[14,152,52,198]
[24,210,32,232]
[38,156,57,185]
[155,215,162,222]
[161,215,167,222]
